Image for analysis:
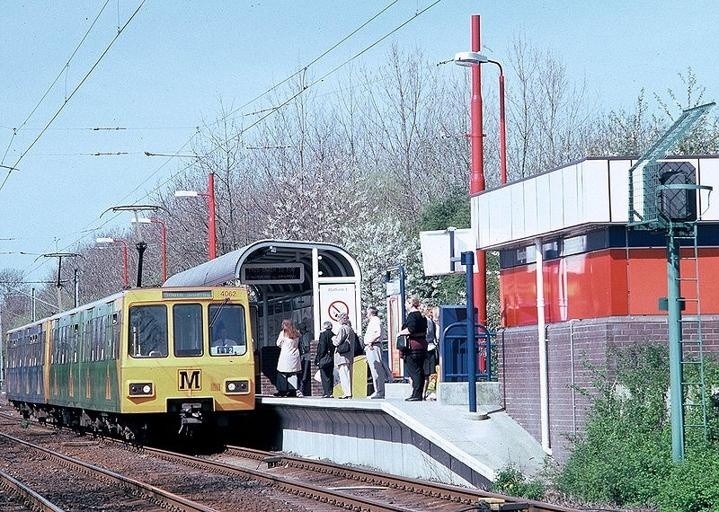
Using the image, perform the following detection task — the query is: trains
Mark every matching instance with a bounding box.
[3,280,260,451]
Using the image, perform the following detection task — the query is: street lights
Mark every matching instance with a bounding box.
[131,217,168,284]
[167,188,217,261]
[453,52,510,185]
[97,237,129,287]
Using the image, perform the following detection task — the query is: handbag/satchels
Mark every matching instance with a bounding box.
[381,361,394,382]
[319,356,333,370]
[336,343,351,354]
[297,336,310,355]
[396,336,407,350]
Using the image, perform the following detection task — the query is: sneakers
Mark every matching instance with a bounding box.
[272,390,438,403]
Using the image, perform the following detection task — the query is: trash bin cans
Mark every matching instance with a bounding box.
[333,355,368,397]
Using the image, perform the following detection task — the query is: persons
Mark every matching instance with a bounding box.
[273,318,302,397]
[329,320,352,397]
[314,320,337,398]
[393,295,440,402]
[212,326,238,349]
[330,312,356,399]
[295,322,314,397]
[363,305,386,400]
